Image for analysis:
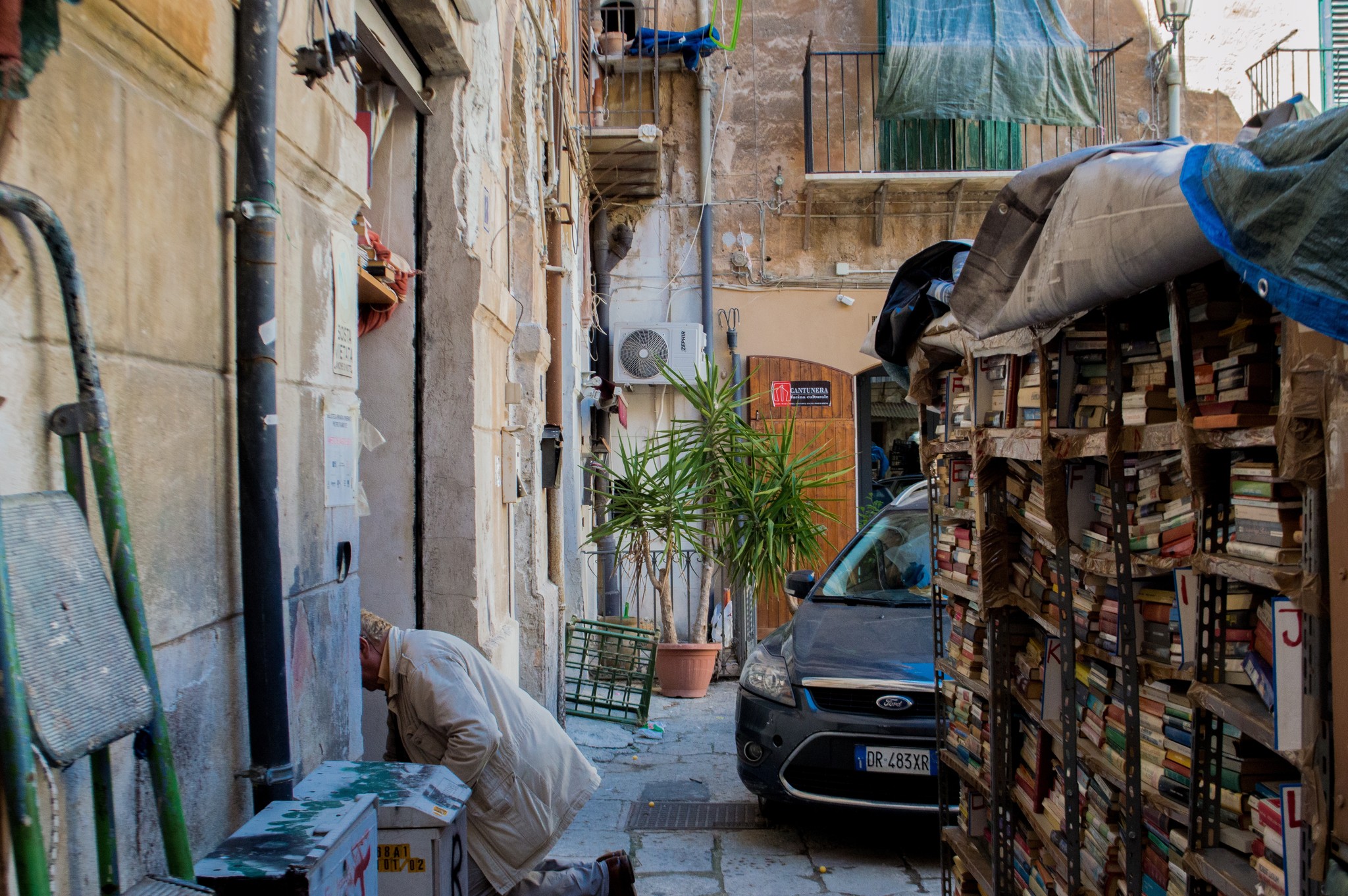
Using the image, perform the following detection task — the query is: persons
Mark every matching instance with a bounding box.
[360,609,636,896]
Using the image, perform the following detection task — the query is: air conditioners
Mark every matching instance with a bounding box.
[608,319,706,390]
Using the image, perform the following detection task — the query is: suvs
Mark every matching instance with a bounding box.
[733,476,965,829]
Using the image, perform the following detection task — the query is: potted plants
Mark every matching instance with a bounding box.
[574,350,861,703]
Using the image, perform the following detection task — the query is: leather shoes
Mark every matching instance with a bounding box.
[596,848,638,896]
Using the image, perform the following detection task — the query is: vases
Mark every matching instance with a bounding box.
[599,30,628,60]
[596,615,642,673]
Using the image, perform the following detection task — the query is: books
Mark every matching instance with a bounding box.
[942,679,1012,785]
[1066,328,1178,428]
[936,522,978,587]
[941,588,1029,685]
[1013,628,1060,702]
[980,354,1056,428]
[1072,573,1182,667]
[1002,449,1196,561]
[1015,720,1227,896]
[1226,462,1302,565]
[956,778,1013,858]
[1213,579,1273,713]
[941,454,991,512]
[1011,531,1085,621]
[1100,668,1284,831]
[951,856,989,896]
[932,358,972,442]
[1059,653,1116,749]
[1247,779,1301,896]
[1189,301,1282,429]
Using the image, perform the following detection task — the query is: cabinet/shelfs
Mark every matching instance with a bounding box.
[911,256,1348,896]
